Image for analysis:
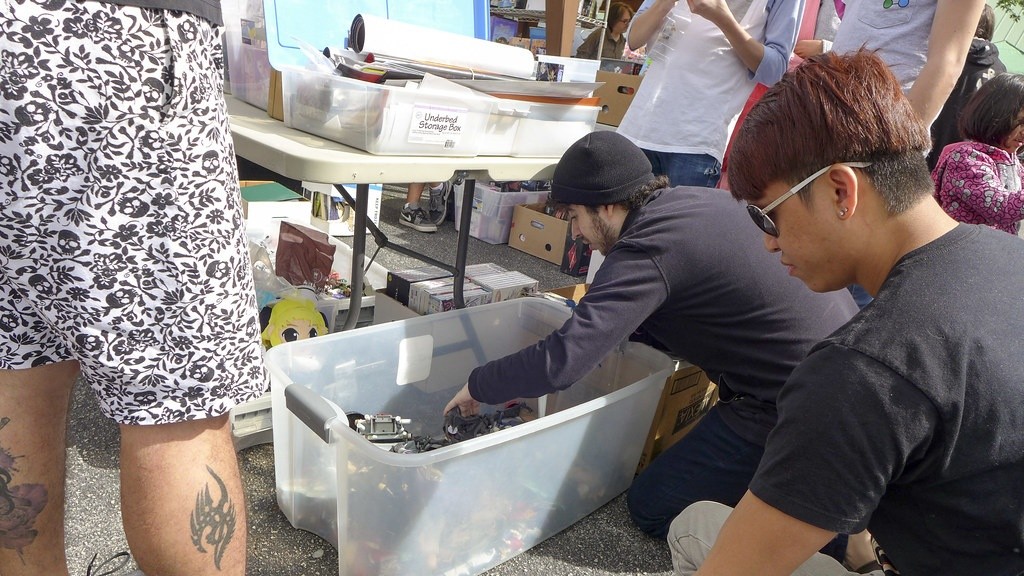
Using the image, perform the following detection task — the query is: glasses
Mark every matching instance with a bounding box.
[746,161,873,239]
[619,20,631,25]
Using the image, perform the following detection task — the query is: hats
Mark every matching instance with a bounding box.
[552,131,655,204]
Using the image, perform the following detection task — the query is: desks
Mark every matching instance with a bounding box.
[224,92,559,382]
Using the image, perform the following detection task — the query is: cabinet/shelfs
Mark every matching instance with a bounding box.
[489,1,611,61]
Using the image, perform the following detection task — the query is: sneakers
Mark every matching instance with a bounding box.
[428,180,452,226]
[399,203,437,232]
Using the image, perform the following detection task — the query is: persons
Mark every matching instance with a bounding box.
[926,2,1008,174]
[668,40,1024,576]
[444,131,901,576]
[931,69,1024,236]
[575,2,634,73]
[615,0,806,188]
[0,1,271,575]
[715,0,850,190]
[831,0,987,130]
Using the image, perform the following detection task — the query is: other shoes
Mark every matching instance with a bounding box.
[854,559,884,574]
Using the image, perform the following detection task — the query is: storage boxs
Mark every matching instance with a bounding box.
[220,0,720,576]
